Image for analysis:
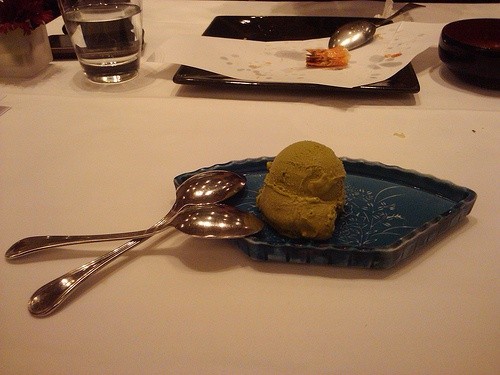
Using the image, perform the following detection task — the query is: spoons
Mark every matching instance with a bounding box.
[5,202,263,260]
[329,3,426,51]
[28,170,248,319]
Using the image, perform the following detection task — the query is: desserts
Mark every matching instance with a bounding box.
[256,140,346,241]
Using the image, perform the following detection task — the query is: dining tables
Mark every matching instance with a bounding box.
[0,0,500,375]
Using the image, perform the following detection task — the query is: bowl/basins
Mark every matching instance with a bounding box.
[438,18,500,80]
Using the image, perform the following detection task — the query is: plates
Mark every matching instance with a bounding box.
[173,156,477,271]
[48,29,145,57]
[171,16,421,92]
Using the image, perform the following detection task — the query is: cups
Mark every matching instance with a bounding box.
[58,0,144,83]
